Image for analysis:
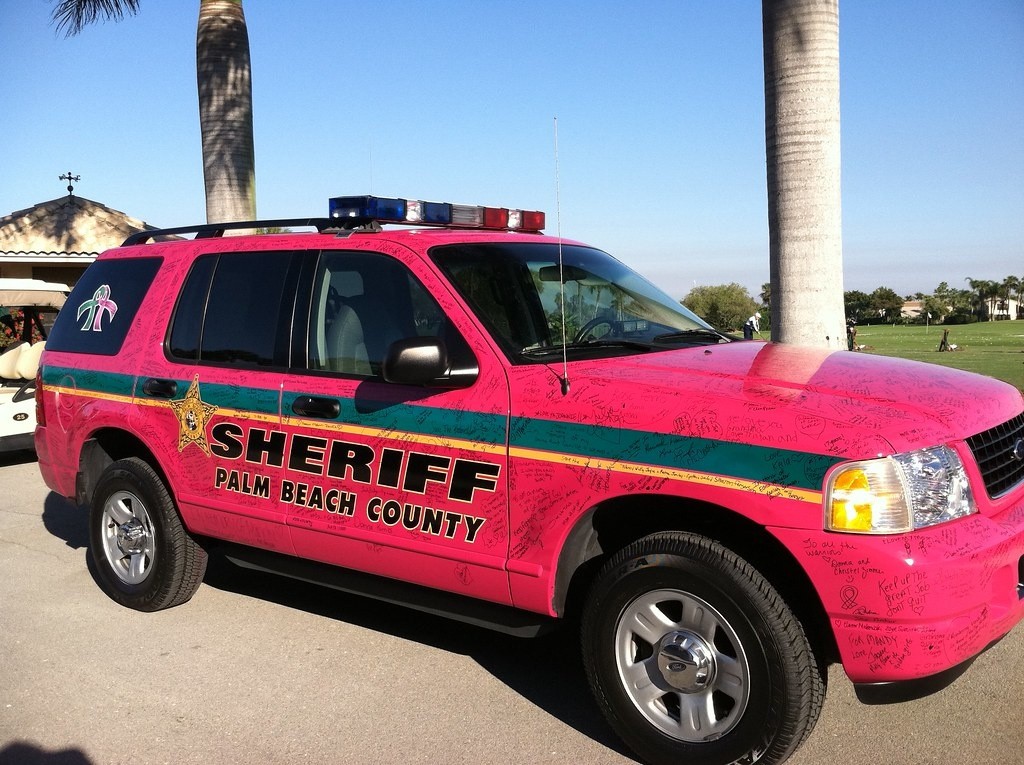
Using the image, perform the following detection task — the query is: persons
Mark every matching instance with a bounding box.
[845,319,875,352]
[939,328,957,351]
[742,313,761,340]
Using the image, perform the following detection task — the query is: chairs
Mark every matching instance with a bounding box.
[327,254,418,376]
[436,263,514,367]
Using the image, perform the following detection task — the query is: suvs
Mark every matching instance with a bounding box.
[33,196,1024,765]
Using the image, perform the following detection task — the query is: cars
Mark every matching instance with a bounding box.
[0,279,70,453]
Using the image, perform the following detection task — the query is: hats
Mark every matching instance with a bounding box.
[755,312,761,318]
